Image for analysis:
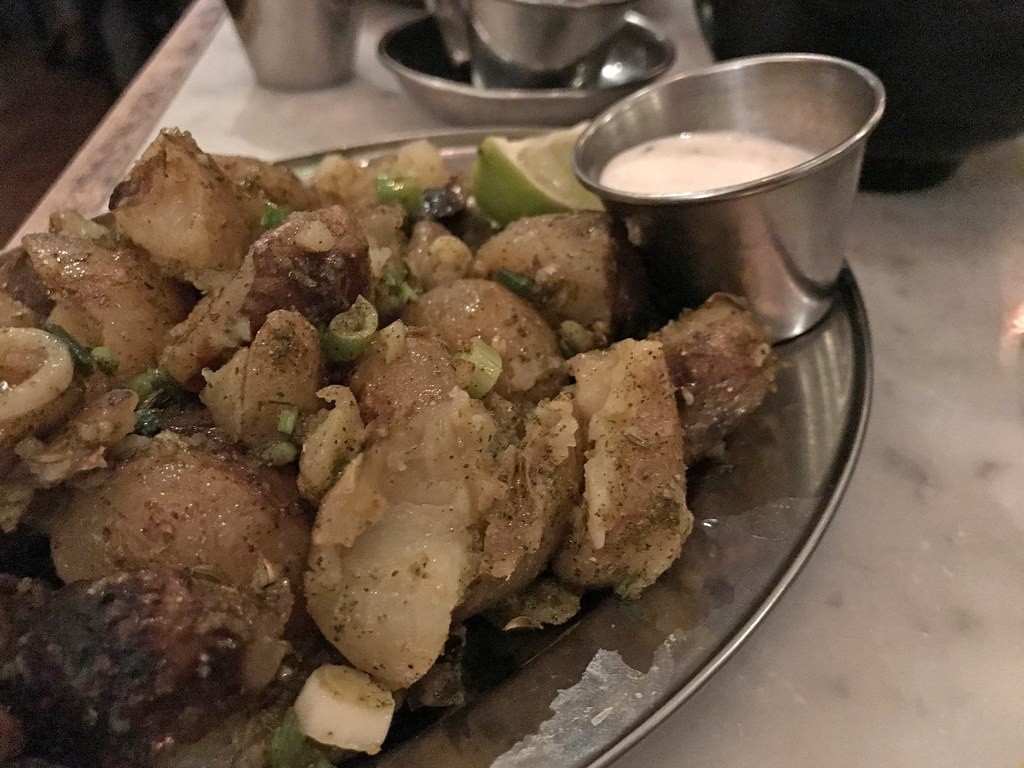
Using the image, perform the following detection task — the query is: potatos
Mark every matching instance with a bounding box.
[1,122,781,768]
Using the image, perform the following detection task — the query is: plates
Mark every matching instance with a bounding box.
[0,0,715,254]
[0,128,873,768]
[375,12,678,127]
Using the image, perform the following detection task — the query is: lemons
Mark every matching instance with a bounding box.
[469,122,608,221]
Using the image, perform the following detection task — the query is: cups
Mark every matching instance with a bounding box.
[221,0,361,96]
[467,0,637,91]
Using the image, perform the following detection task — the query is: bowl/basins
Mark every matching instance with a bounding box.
[570,53,886,342]
